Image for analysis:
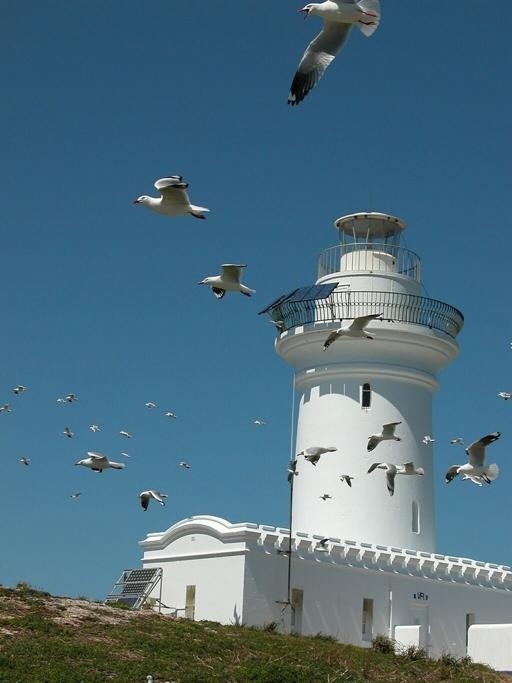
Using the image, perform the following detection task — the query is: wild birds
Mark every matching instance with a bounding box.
[0,385,176,474]
[268,320,284,328]
[180,461,191,470]
[287,0,381,107]
[254,418,268,427]
[499,392,512,400]
[139,490,167,511]
[70,493,82,501]
[131,174,211,221]
[323,312,384,352]
[284,421,502,501]
[196,263,256,299]
